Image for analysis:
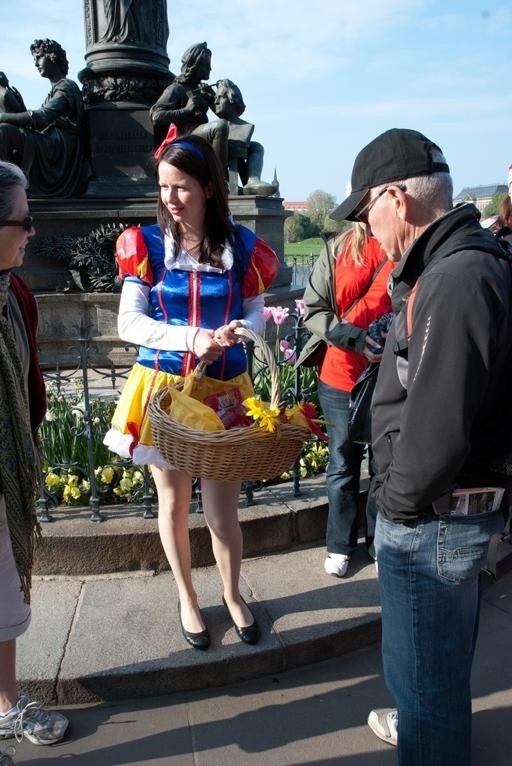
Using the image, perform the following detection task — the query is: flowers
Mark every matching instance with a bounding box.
[242,396,318,433]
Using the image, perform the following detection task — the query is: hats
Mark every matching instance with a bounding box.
[329,128,449,222]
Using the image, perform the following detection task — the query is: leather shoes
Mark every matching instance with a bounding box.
[222,593,260,644]
[177,599,210,650]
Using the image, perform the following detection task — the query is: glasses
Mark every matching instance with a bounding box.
[355,184,405,224]
[0,217,33,229]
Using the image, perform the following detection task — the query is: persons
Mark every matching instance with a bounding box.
[101,133,282,653]
[0,161,78,750]
[0,72,27,114]
[286,219,401,579]
[213,75,254,159]
[147,36,275,198]
[327,125,512,766]
[1,36,88,196]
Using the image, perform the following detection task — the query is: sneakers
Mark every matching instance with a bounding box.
[0,696,71,745]
[367,707,398,746]
[324,553,350,577]
